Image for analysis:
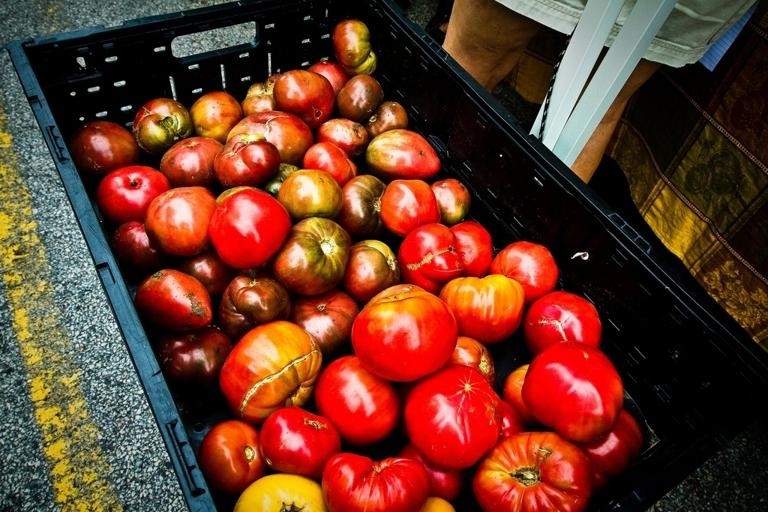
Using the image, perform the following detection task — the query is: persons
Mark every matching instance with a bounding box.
[442,1,757,186]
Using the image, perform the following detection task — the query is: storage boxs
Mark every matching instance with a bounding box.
[9,1,767,512]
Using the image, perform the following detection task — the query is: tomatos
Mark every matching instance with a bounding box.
[69,19,642,512]
